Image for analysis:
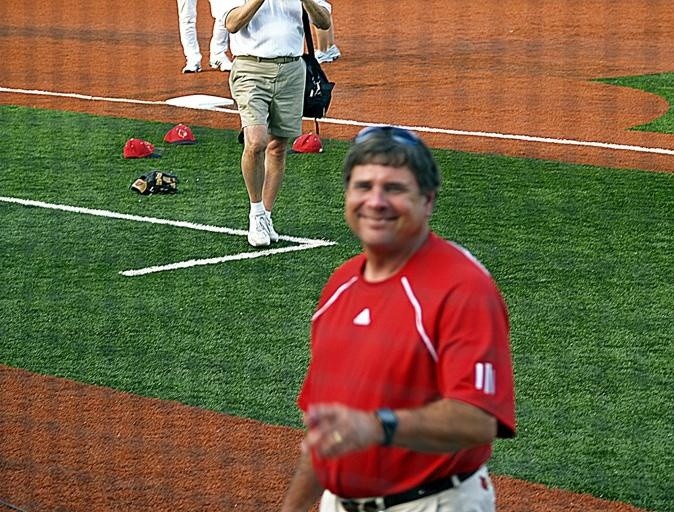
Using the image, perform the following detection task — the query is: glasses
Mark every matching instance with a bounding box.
[353,124,418,147]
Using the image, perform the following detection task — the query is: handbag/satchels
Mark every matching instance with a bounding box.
[300,4,335,119]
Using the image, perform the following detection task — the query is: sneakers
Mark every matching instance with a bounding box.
[248,210,279,248]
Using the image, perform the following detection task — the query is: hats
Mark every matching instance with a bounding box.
[162,123,198,146]
[123,137,164,159]
[287,131,323,154]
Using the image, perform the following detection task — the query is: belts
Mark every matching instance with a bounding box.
[232,52,302,65]
[339,469,479,512]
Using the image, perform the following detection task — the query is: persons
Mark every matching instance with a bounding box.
[311,0,341,65]
[175,0,231,76]
[279,125,518,511]
[220,0,331,251]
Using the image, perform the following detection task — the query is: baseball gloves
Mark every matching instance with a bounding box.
[130,170,178,196]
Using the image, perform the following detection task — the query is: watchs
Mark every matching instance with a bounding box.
[374,407,399,447]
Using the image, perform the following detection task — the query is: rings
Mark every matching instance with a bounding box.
[331,430,342,445]
